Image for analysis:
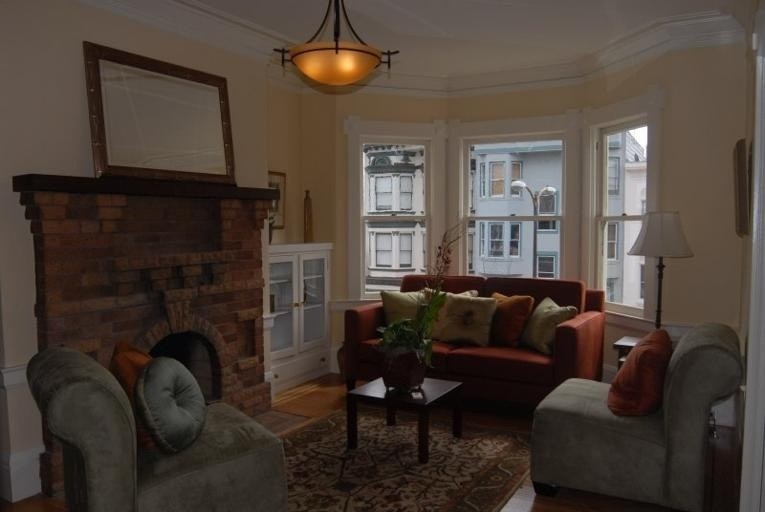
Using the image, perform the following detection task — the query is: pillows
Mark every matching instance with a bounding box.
[379,288,426,332]
[606,326,675,417]
[447,290,480,300]
[135,355,207,454]
[420,287,499,349]
[109,345,155,452]
[520,297,579,357]
[489,292,536,349]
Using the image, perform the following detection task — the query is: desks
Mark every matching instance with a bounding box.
[610,335,642,370]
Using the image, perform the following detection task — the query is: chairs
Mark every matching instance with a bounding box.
[527,320,743,510]
[23,346,290,510]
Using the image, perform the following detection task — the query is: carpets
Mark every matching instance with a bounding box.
[250,411,311,437]
[272,392,533,512]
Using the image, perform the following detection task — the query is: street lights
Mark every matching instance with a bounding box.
[510,181,558,278]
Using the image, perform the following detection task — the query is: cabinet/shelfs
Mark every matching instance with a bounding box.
[260,243,336,397]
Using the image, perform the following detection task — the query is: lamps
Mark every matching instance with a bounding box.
[271,0,400,86]
[628,209,696,329]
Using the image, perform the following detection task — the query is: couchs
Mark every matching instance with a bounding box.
[336,274,606,416]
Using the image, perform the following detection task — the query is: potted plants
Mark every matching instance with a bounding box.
[372,214,476,399]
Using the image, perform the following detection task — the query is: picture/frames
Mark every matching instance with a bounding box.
[83,40,237,182]
[266,170,288,230]
[732,138,751,238]
